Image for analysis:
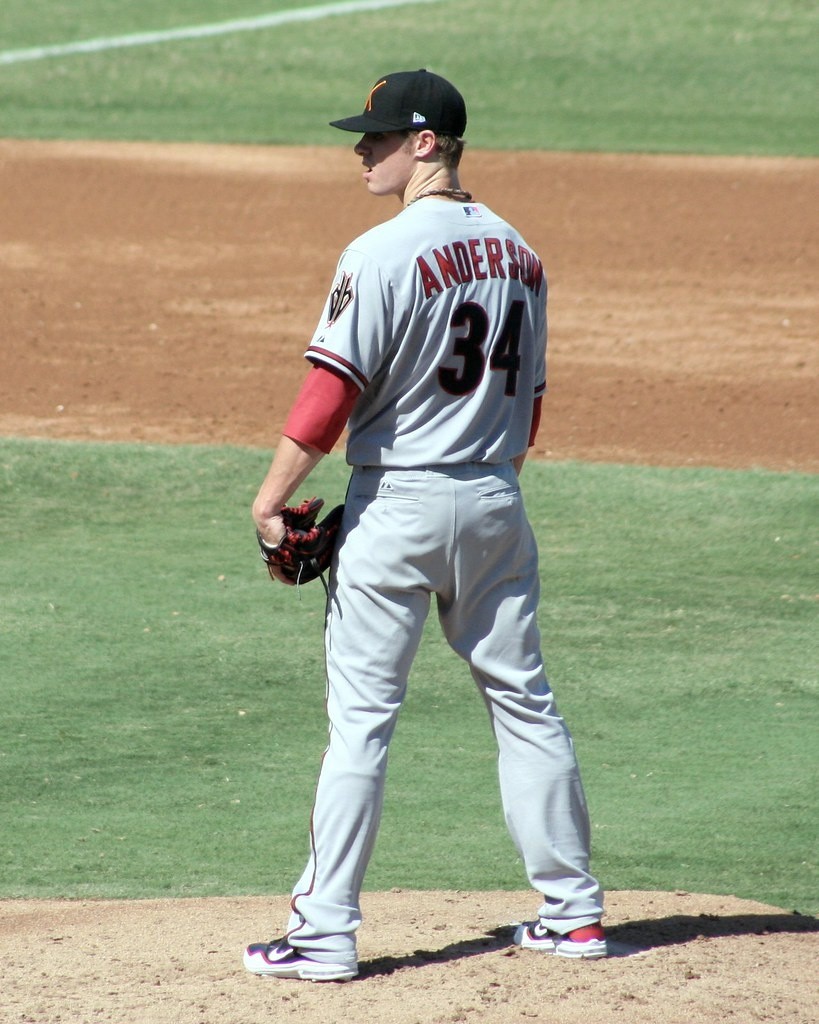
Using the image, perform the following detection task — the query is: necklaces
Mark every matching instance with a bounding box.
[406,188,473,203]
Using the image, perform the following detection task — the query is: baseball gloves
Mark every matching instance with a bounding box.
[253,495,346,603]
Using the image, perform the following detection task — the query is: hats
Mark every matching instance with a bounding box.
[328,68,468,138]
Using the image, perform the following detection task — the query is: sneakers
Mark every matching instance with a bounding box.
[512,922,607,960]
[243,937,359,984]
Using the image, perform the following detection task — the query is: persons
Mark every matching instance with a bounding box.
[245,69,609,981]
